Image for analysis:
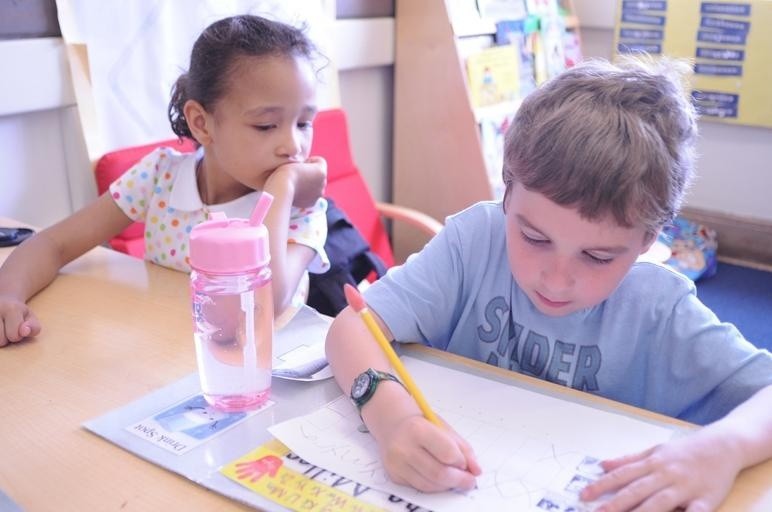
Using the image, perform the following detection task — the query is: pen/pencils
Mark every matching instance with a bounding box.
[345,281,480,494]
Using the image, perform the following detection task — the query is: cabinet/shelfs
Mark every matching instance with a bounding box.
[390,0,584,266]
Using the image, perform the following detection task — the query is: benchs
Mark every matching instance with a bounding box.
[92,106,443,282]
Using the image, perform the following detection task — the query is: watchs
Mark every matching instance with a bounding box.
[348,367,413,426]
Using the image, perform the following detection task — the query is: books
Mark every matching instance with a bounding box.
[443,0,590,205]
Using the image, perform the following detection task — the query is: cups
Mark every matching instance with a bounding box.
[189,215,276,411]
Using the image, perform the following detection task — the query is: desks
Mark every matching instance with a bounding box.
[1,207,772,509]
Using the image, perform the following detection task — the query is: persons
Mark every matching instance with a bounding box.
[322,48,771,512]
[0,14,331,351]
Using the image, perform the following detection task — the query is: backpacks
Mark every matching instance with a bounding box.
[308,196,388,317]
